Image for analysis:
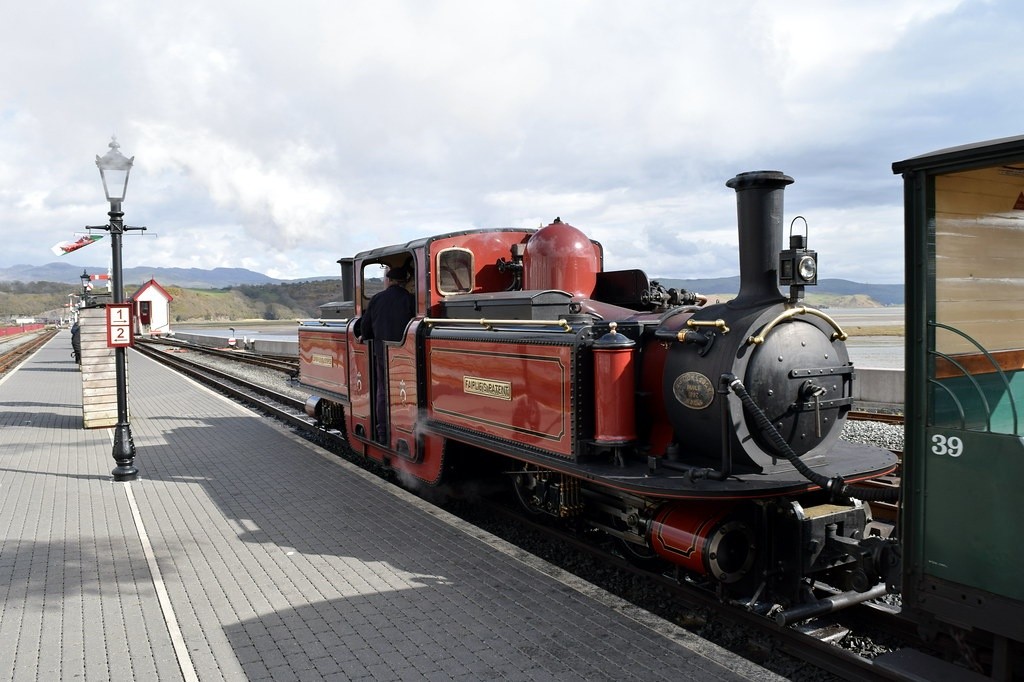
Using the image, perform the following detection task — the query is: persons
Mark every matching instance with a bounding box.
[358,267,416,344]
[71,322,81,371]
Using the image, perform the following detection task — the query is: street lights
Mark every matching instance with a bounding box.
[84,133,147,483]
[80,270,91,306]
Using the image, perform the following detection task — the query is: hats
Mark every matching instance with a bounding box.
[386,266,408,279]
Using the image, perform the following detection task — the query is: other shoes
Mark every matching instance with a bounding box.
[379,433,387,443]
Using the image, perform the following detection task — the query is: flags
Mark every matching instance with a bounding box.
[51,234,106,257]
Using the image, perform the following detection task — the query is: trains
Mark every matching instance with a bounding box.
[296,134,1024,682]
